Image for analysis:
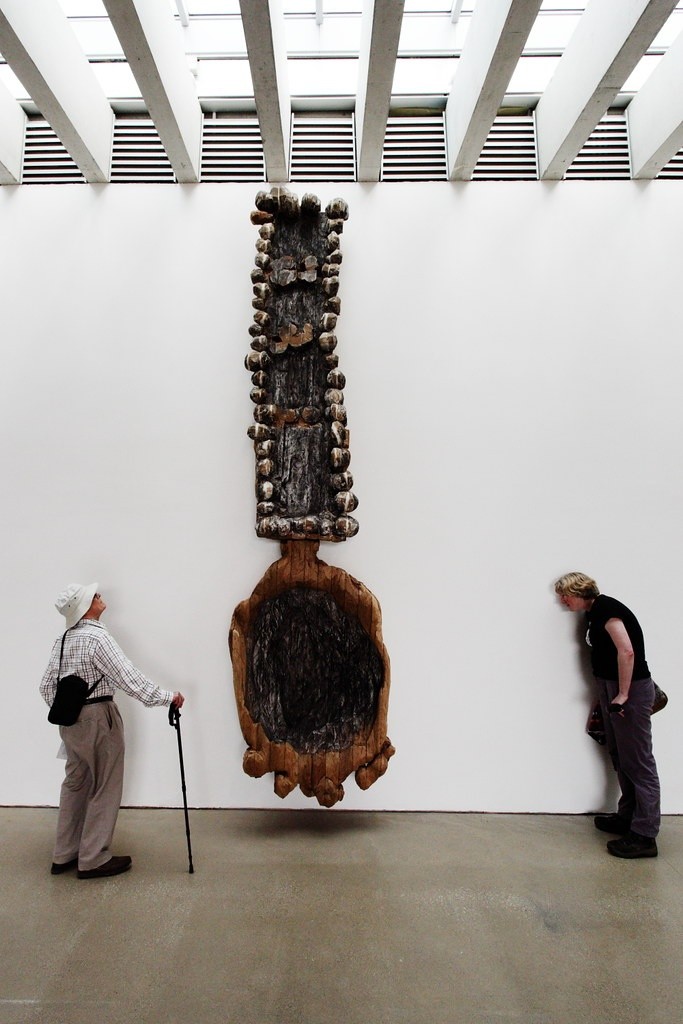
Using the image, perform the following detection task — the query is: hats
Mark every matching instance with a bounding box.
[55,584,99,629]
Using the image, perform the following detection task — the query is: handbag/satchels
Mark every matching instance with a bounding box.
[649,682,668,715]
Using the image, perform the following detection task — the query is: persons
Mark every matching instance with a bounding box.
[38,579,184,879]
[553,573,663,858]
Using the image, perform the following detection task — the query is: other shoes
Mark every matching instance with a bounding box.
[51,858,78,874]
[78,856,132,879]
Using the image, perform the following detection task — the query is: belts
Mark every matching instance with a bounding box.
[85,695,113,705]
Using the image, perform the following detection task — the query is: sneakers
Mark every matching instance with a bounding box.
[594,813,631,835]
[607,829,658,858]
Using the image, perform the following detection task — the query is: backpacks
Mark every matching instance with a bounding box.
[48,675,89,726]
